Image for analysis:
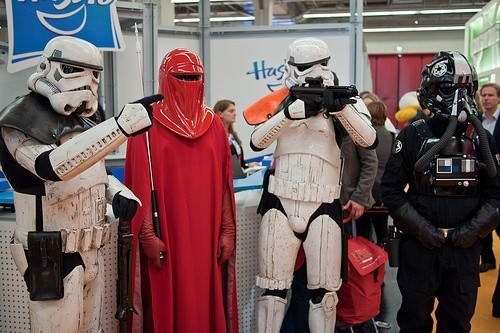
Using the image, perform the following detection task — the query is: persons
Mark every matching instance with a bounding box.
[212,99,262,177]
[124,47,241,333]
[475,83,500,272]
[0,35,165,333]
[378,49,500,333]
[342,102,397,244]
[243,37,379,333]
[361,94,399,139]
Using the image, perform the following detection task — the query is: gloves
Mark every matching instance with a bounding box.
[141,192,167,268]
[394,203,443,251]
[284,98,322,120]
[452,202,500,250]
[327,99,345,112]
[115,94,164,137]
[112,191,137,220]
[217,189,235,264]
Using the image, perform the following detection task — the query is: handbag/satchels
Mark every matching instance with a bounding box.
[337,236,388,324]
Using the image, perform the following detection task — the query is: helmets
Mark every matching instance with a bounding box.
[416,51,478,118]
[151,48,215,138]
[285,36,334,89]
[27,35,104,117]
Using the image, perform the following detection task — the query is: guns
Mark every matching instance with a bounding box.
[289,76,358,116]
